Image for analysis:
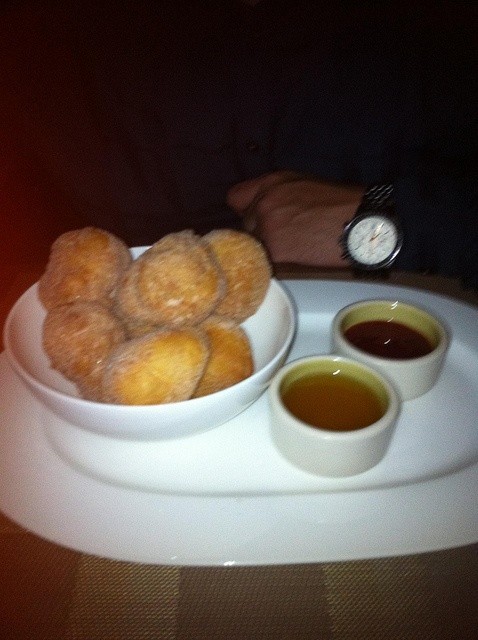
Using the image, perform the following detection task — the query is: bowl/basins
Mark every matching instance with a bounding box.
[330,297,449,403]
[272,355,401,475]
[4,242,298,442]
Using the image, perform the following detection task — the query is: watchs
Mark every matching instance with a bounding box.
[338,183,406,282]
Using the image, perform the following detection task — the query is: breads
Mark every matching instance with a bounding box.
[202,227,274,321]
[190,315,255,399]
[38,225,133,311]
[41,300,126,398]
[98,324,210,404]
[114,227,225,343]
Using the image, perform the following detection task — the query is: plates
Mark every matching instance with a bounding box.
[1,278,478,571]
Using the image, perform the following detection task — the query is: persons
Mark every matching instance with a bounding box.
[223,168,478,291]
